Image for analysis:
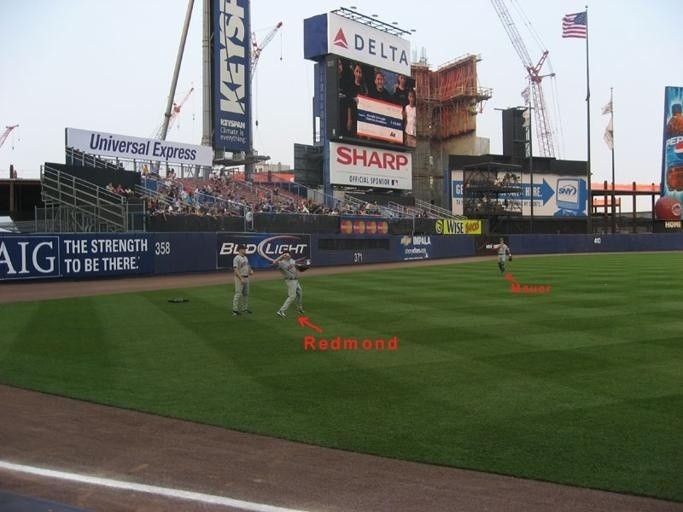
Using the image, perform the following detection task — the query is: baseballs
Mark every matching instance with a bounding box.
[286,253,290,257]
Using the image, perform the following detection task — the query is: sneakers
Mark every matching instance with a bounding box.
[232,311,241,315]
[276,312,286,317]
[297,310,305,313]
[243,310,251,313]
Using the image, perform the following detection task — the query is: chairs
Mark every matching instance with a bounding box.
[173,170,336,214]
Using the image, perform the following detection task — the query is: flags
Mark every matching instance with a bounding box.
[562,12,586,37]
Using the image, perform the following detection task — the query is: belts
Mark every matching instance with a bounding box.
[290,278,296,280]
[244,275,248,277]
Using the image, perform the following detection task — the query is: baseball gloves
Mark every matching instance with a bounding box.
[509,256,512,261]
[296,264,309,272]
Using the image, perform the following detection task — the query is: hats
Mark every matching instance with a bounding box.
[237,245,246,249]
[283,250,290,253]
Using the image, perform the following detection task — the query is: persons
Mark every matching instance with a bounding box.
[337,57,416,148]
[232,244,254,316]
[273,250,312,319]
[485,237,512,275]
